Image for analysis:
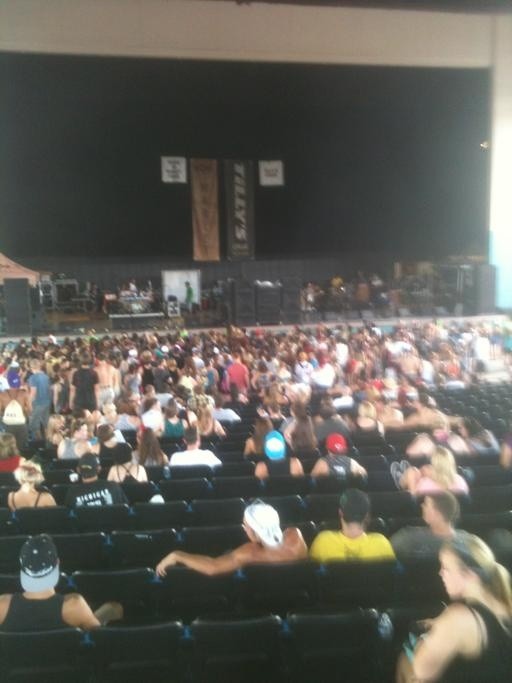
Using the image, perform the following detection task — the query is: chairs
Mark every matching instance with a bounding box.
[0,377,512,683]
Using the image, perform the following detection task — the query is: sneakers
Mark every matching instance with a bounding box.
[390,462,402,492]
[400,459,410,474]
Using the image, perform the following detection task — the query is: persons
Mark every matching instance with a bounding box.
[309,488,396,561]
[389,491,458,556]
[155,503,308,578]
[396,534,512,683]
[1,277,512,511]
[0,533,123,631]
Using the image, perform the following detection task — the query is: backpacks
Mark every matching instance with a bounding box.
[297,362,310,385]
[3,390,27,426]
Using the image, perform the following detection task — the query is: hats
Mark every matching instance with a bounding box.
[20,533,60,593]
[7,372,20,387]
[244,503,284,547]
[264,431,286,460]
[327,433,348,453]
[79,453,98,479]
